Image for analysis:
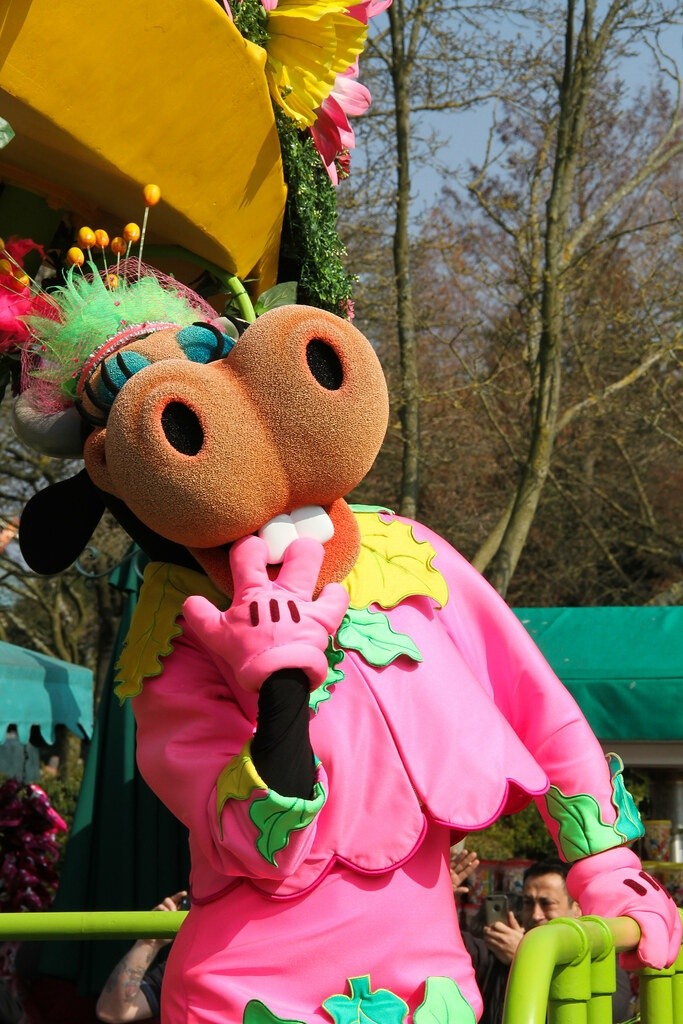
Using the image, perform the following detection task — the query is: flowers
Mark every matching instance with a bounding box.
[219,0,392,325]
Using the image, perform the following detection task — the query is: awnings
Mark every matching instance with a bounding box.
[0,634,97,743]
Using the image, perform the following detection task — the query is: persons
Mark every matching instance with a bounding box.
[443,847,635,1024]
[96,890,196,1024]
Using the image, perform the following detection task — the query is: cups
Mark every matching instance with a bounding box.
[642,821,672,862]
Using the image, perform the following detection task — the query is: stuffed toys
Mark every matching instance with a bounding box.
[0,189,682,1024]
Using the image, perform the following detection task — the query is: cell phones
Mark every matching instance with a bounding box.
[181,895,192,913]
[484,894,509,927]
